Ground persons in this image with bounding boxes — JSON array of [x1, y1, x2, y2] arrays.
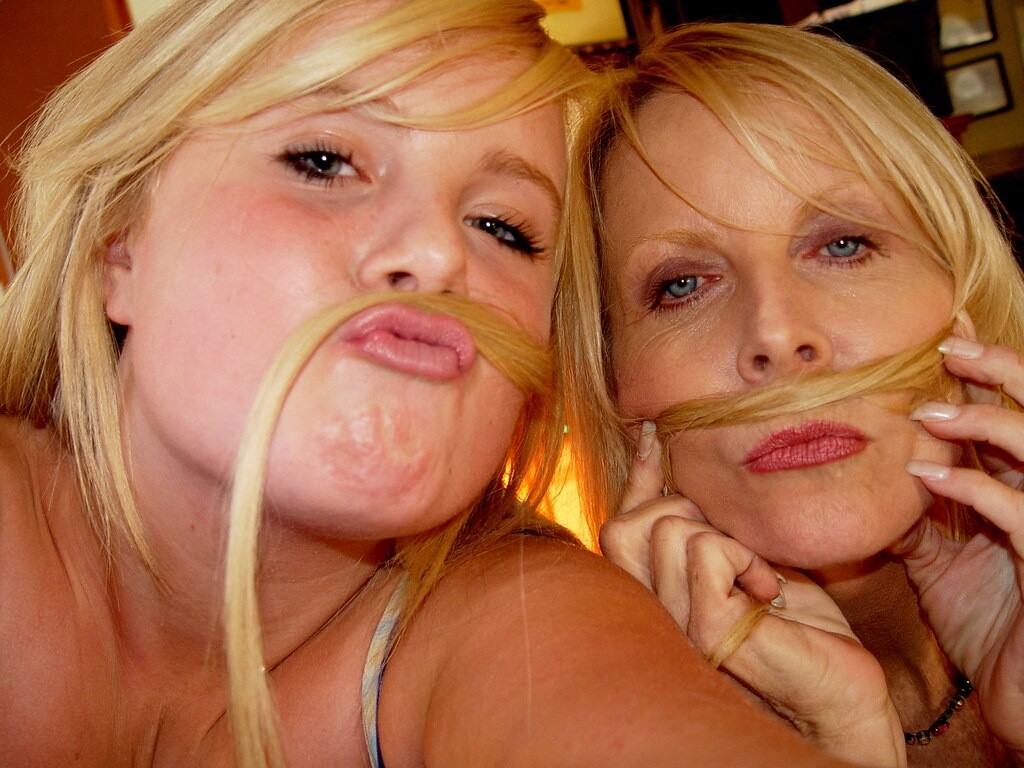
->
[[0, 0, 864, 767], [552, 22, 1024, 767]]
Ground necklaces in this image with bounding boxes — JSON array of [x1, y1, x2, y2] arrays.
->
[[904, 678, 972, 747]]
